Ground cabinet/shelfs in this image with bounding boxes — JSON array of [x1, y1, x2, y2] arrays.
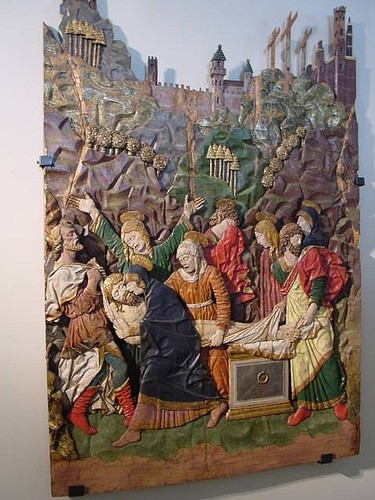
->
[[225, 353, 294, 421]]
